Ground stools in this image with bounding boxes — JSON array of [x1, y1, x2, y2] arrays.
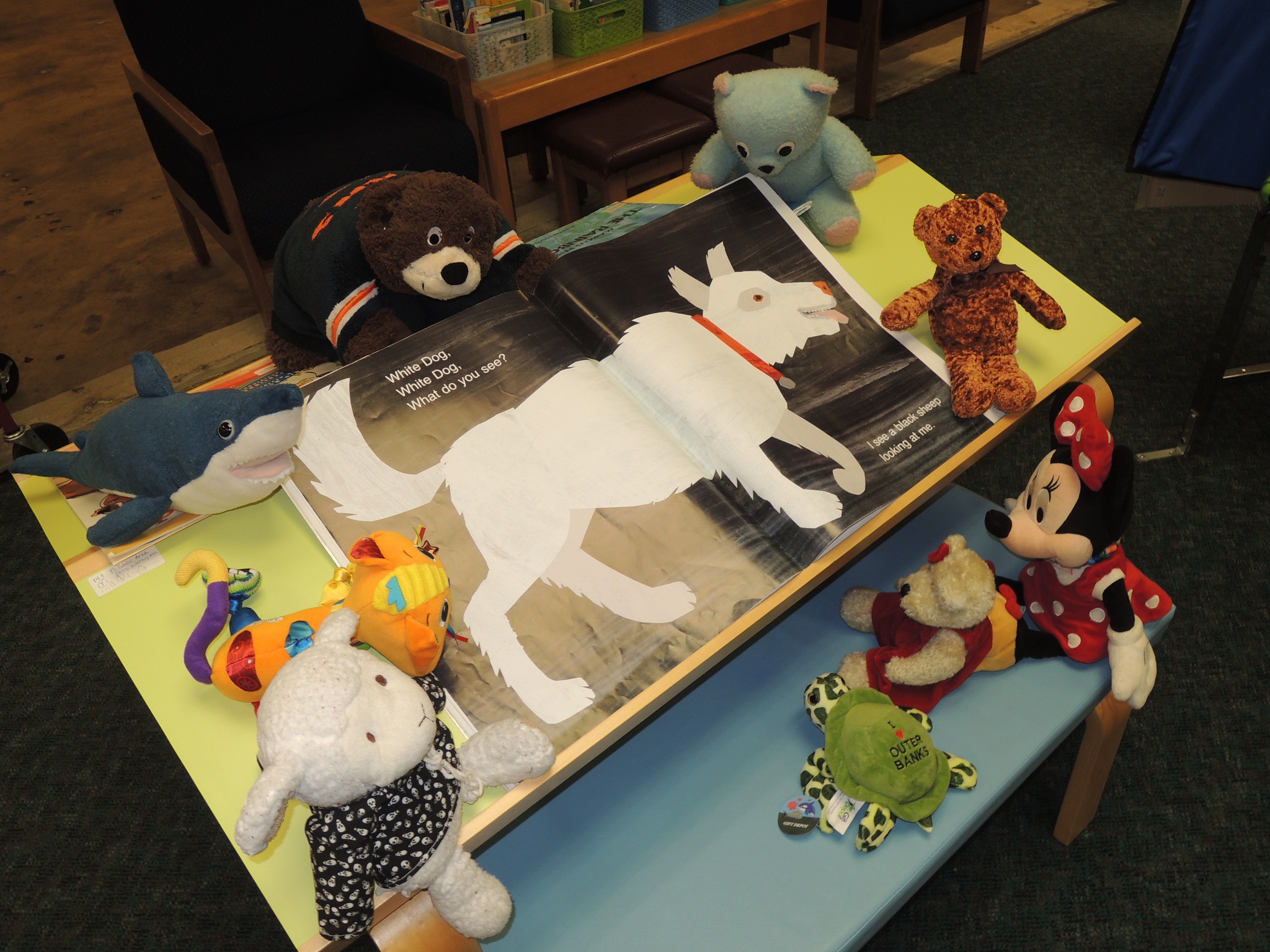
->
[[654, 53, 786, 121], [546, 87, 713, 228]]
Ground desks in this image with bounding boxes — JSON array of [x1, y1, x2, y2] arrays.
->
[[11, 154, 1150, 952], [473, 0, 828, 242]]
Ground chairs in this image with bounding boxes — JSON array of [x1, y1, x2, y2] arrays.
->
[[791, 0, 991, 122], [113, 0, 491, 327]]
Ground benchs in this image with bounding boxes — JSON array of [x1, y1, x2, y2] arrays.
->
[[477, 481, 1175, 951]]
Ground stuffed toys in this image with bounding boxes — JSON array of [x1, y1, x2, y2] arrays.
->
[[973, 383, 1157, 711], [237, 608, 557, 940], [172, 528, 453, 712], [263, 167, 561, 374], [800, 673, 980, 849], [832, 533, 996, 715], [687, 67, 873, 247], [881, 191, 1066, 420], [8, 349, 301, 546]]
